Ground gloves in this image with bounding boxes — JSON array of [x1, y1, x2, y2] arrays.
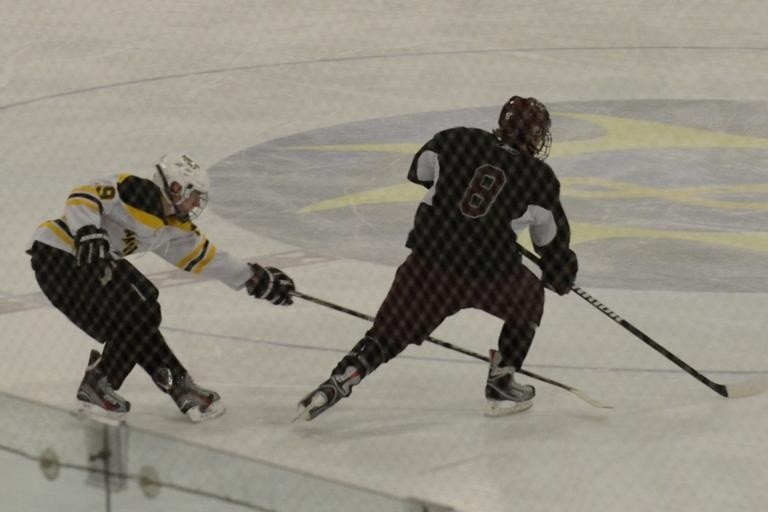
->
[[245, 261, 295, 306], [73, 223, 112, 269], [538, 249, 578, 294]]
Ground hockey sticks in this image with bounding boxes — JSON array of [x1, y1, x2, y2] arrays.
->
[[292, 291, 614, 409], [520, 242, 767, 399]]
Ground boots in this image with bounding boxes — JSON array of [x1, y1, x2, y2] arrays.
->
[[485, 349, 535, 403], [77, 349, 130, 413], [296, 365, 361, 422], [150, 366, 220, 415]]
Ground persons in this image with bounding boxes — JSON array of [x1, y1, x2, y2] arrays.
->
[[26, 149, 294, 414], [297, 94, 579, 420]]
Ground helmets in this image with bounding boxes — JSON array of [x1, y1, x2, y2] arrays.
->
[[153, 151, 209, 224], [497, 96, 552, 162]]
